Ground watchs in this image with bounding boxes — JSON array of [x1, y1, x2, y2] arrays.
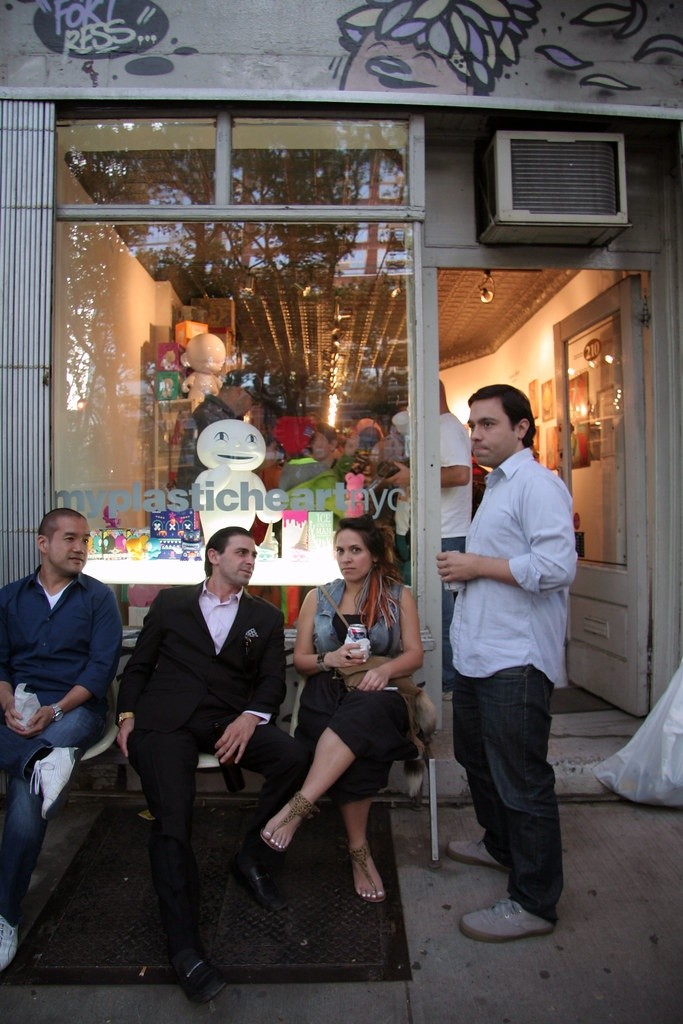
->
[[50, 704, 63, 721], [118, 712, 134, 725]]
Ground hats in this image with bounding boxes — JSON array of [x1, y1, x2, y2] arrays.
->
[[315, 422, 337, 443], [222, 369, 274, 401]]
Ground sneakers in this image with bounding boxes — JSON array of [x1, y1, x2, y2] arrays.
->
[[0, 914, 19, 972], [29, 745, 80, 820]]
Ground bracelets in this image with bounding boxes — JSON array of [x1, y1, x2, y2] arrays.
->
[[317, 652, 331, 671]]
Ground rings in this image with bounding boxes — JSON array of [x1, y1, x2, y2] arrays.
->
[[346, 652, 352, 660]]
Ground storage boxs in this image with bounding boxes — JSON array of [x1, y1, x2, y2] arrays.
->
[[208, 327, 230, 375], [156, 342, 187, 375], [208, 298, 235, 335], [175, 321, 209, 349], [156, 371, 186, 400]]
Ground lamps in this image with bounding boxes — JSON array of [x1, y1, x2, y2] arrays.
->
[[478, 271, 495, 304], [386, 286, 400, 297], [244, 275, 254, 292], [325, 303, 353, 395], [292, 282, 311, 296]]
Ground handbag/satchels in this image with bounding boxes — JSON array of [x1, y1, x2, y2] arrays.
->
[[337, 654, 405, 691]]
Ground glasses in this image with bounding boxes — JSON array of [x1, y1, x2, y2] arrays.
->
[[245, 387, 260, 407]]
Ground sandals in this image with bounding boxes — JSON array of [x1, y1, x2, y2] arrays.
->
[[345, 840, 386, 904], [260, 791, 321, 853]]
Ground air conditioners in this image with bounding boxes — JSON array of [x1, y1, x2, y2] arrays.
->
[[474, 130, 629, 247]]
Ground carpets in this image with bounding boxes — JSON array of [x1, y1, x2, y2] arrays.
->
[[0, 797, 416, 986]]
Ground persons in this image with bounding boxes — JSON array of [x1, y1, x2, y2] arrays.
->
[[253, 415, 384, 628], [182, 333, 226, 410], [435, 385, 575, 941], [385, 380, 473, 694], [115, 528, 299, 1006], [174, 368, 263, 510], [0, 508, 123, 970], [260, 517, 424, 903]]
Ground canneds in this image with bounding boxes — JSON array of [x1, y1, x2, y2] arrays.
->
[[348, 624, 367, 642], [443, 550, 466, 590]]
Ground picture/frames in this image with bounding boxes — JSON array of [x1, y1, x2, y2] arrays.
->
[[529, 370, 592, 472]]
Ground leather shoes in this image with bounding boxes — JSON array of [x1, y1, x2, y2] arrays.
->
[[232, 852, 288, 912], [170, 948, 226, 1005]]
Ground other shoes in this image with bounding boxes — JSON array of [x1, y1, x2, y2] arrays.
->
[[446, 839, 515, 873], [459, 897, 553, 943]]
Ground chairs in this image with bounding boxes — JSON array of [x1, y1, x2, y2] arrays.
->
[[0, 665, 442, 870]]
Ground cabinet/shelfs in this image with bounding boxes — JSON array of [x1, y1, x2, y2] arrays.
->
[[155, 399, 198, 492]]
